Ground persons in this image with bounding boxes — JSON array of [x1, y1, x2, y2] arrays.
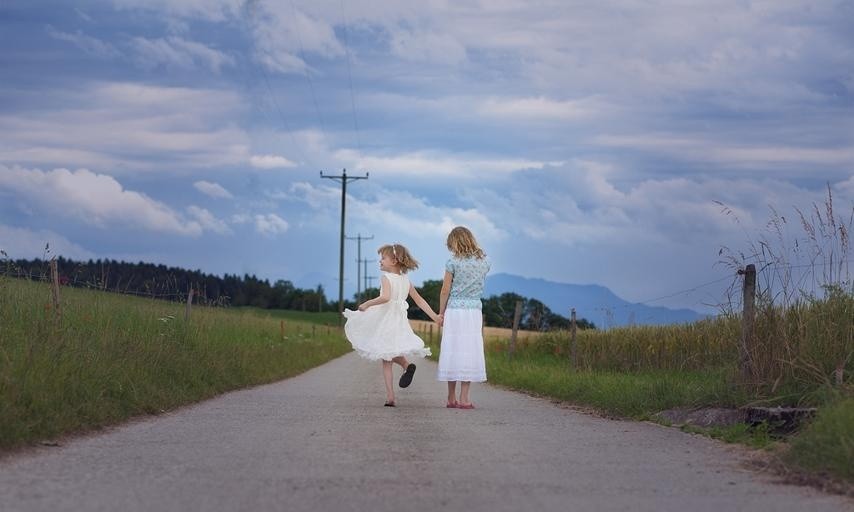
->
[[341, 244, 444, 407], [438, 226, 491, 409]]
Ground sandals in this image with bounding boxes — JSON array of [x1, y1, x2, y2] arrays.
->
[[398, 363, 416, 388], [384, 401, 395, 406], [447, 399, 475, 409]]
